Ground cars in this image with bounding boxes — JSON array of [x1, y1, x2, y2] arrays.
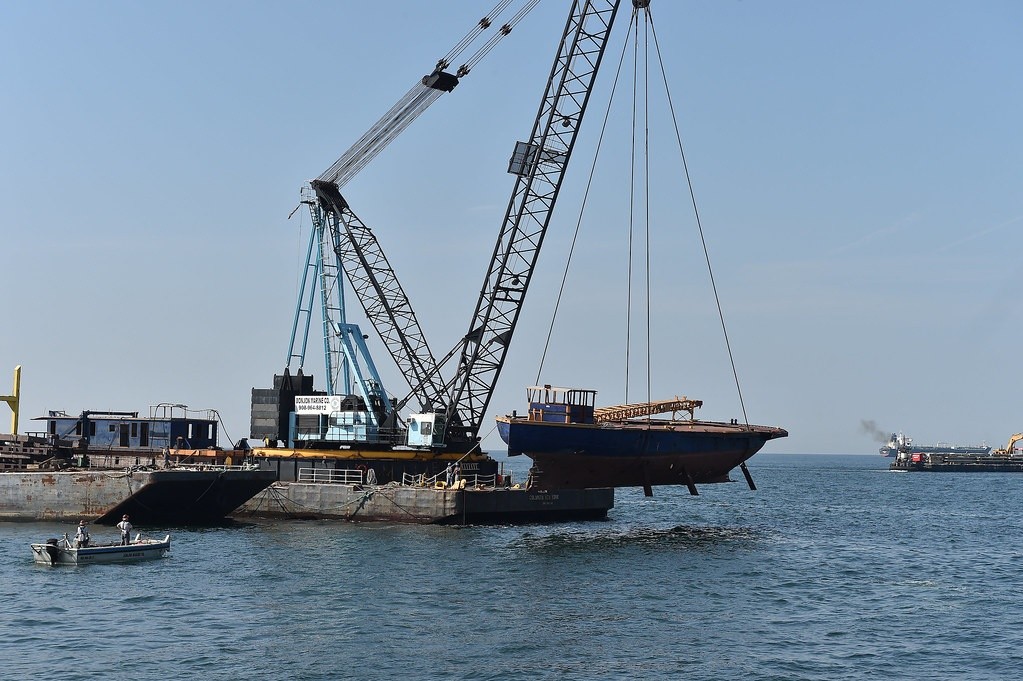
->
[[131, 463, 228, 473]]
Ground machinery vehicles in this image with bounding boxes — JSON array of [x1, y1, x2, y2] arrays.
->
[[991, 431, 1023, 457], [279, 1, 654, 452]]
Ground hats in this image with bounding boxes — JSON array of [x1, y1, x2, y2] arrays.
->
[[122, 515, 129, 521], [79, 520, 85, 525]]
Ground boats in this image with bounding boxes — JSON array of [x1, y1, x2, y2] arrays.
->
[[31, 532, 170, 567], [496, 384, 788, 490], [878, 430, 993, 458]]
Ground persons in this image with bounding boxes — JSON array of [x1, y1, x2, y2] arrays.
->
[[446, 462, 461, 488], [262, 434, 270, 448], [117, 514, 133, 547], [164, 445, 172, 469], [76, 519, 90, 548]]
